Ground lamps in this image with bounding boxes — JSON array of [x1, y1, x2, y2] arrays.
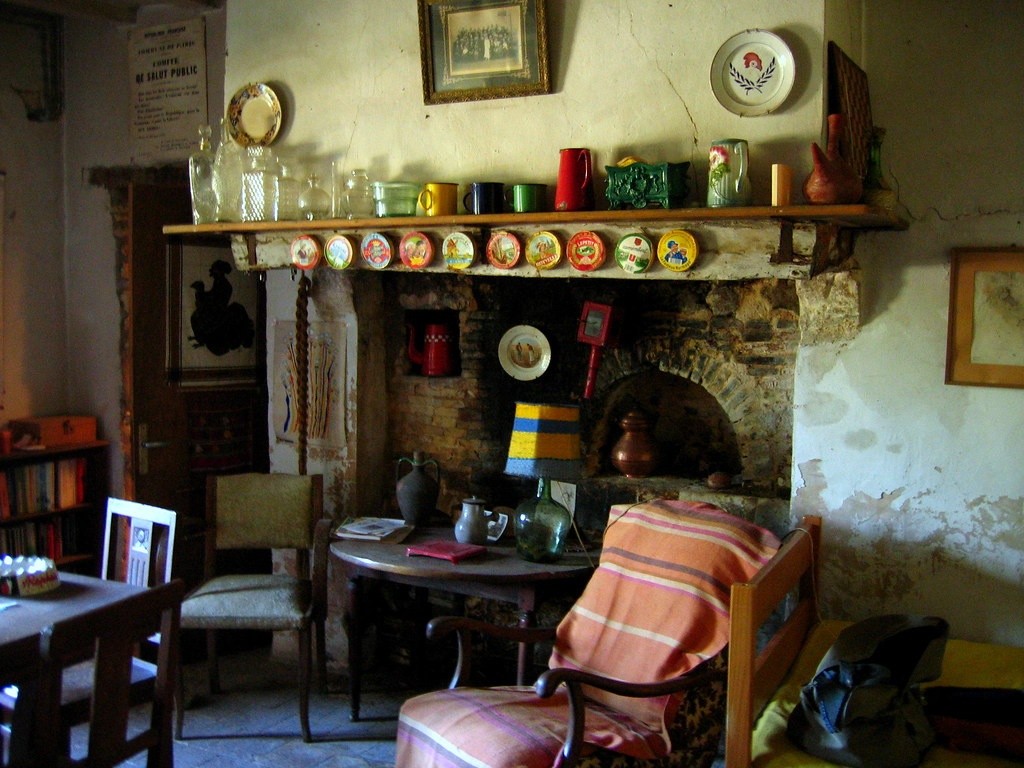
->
[[502, 402, 583, 563]]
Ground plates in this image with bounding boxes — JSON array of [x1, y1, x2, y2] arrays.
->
[[498, 324, 551, 381], [226, 83, 282, 148], [709, 28, 796, 118]]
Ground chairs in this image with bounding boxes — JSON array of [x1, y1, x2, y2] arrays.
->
[[0, 497, 183, 768], [394, 499, 782, 768], [176, 474, 325, 743]]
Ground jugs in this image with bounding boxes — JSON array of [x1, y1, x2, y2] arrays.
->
[[406, 324, 455, 377], [553, 148, 594, 211]]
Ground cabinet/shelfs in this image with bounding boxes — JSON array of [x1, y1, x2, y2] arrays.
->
[[0, 439, 113, 580]]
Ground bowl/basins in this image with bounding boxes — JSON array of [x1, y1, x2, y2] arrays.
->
[[371, 181, 419, 218]]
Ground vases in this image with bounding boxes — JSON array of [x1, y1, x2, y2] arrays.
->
[[340, 169, 375, 219]]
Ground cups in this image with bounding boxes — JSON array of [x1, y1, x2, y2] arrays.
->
[[419, 182, 459, 216], [506, 183, 547, 212], [707, 138, 750, 208], [462, 182, 504, 214]]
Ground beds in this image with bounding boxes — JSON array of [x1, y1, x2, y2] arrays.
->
[[725, 514, 1024, 768]]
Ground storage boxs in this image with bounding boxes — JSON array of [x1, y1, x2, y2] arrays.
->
[[9, 414, 97, 446]]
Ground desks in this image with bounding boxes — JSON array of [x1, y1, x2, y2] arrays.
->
[[0, 570, 158, 692], [327, 520, 601, 723]]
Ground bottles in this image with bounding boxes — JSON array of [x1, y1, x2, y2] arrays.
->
[[189, 125, 216, 225], [612, 407, 656, 477], [455, 495, 487, 545], [395, 446, 440, 529], [299, 161, 374, 220], [241, 145, 279, 222], [275, 164, 300, 220], [211, 118, 243, 223]]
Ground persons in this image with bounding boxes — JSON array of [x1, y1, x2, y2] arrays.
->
[[454, 25, 512, 61]]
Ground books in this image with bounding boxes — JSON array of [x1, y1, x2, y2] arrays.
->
[[336, 516, 381, 540], [0, 459, 85, 560]]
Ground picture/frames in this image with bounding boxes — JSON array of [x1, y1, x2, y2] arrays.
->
[[416, 0, 553, 107], [943, 244, 1024, 389]]
